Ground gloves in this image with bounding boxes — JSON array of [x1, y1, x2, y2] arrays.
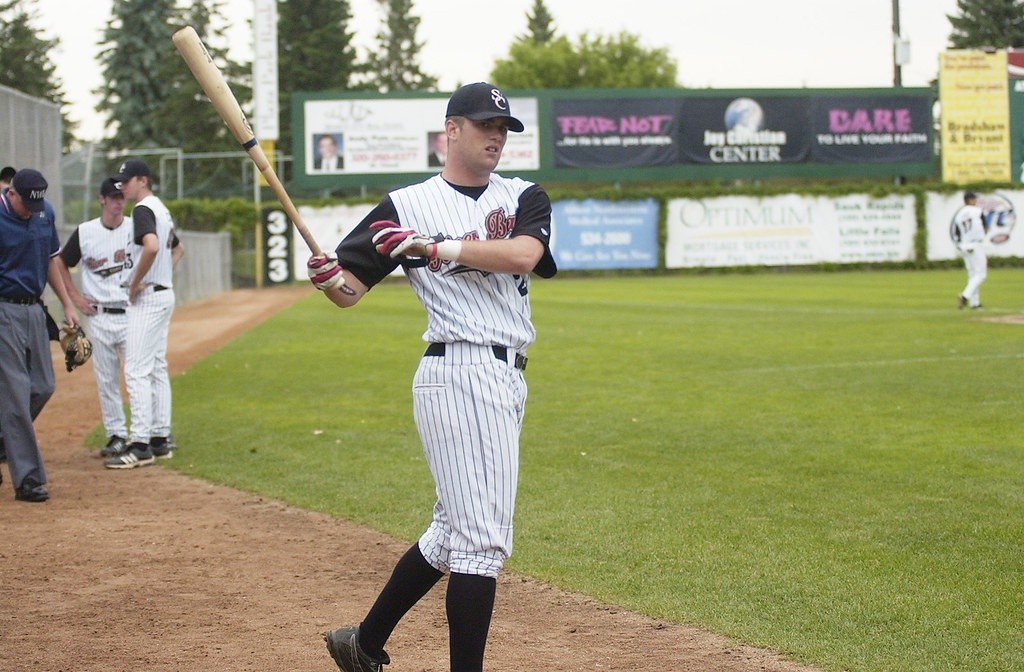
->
[[307, 253, 345, 291], [369, 219, 438, 259]]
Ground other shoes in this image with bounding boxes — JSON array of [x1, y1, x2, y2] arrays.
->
[[970, 304, 983, 310], [15, 477, 49, 501], [958, 294, 968, 309]]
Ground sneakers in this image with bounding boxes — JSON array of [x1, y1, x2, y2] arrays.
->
[[150, 439, 176, 460], [105, 442, 154, 469], [321, 621, 390, 672], [101, 435, 126, 457]]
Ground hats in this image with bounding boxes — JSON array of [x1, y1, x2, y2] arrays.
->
[[101, 178, 123, 198], [13, 168, 48, 212], [110, 159, 151, 182], [446, 82, 524, 133]]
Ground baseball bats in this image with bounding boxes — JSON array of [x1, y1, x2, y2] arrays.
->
[[163, 21, 332, 266]]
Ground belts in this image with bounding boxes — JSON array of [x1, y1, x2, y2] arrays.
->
[[424, 342, 528, 370], [0, 295, 41, 306], [154, 285, 168, 292], [91, 305, 125, 314]]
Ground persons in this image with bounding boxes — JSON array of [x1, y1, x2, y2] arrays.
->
[[428, 132, 448, 167], [314, 135, 344, 169], [0, 166, 80, 502], [307, 82, 558, 672], [103, 160, 187, 470], [56, 176, 178, 458], [950, 192, 989, 310]]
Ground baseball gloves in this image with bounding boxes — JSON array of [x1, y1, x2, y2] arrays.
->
[[58, 320, 92, 365]]
